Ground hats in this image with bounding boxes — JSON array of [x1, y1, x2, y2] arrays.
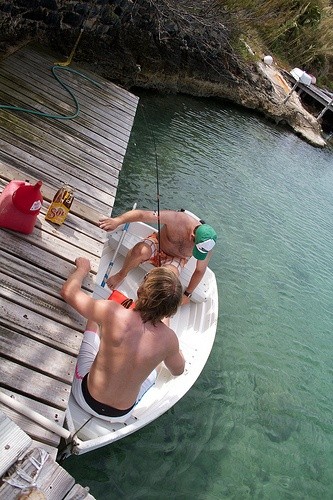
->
[[192, 224, 217, 260]]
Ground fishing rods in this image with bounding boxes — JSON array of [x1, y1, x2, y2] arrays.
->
[[138, 102, 162, 267]]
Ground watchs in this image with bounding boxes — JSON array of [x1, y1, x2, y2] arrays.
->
[[183, 290, 193, 299]]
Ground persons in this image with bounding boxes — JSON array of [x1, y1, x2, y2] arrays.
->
[[98, 208, 217, 307], [61, 256, 187, 424]]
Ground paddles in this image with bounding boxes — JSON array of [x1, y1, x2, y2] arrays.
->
[[100, 201, 138, 288]]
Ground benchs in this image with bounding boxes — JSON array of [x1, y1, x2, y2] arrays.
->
[[108, 230, 210, 304], [68, 389, 140, 442]]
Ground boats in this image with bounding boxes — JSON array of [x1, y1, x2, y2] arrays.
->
[[47, 215, 220, 457]]
